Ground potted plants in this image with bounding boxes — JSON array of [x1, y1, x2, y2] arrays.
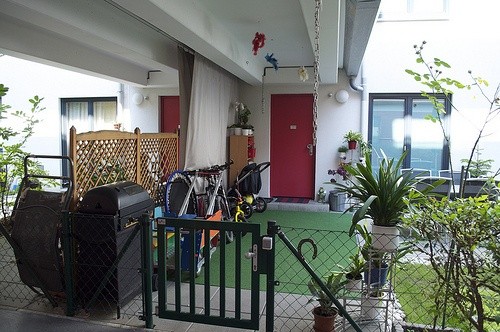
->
[[303, 144, 446, 332], [343, 130, 362, 149], [338, 146, 349, 158], [227, 122, 254, 136]]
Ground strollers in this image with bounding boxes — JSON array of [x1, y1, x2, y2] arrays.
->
[[224, 161, 272, 219]]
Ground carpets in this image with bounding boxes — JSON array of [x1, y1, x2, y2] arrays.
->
[[150, 208, 364, 301]]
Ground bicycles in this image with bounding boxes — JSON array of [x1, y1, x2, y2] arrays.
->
[[180, 159, 236, 278]]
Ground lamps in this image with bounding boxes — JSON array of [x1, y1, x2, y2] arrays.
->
[[335, 89, 349, 104], [132, 93, 143, 104]]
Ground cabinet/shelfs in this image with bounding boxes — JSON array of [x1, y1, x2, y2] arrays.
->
[[227, 135, 254, 190]]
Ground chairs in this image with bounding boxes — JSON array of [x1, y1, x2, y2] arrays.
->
[[400, 168, 498, 212]]
